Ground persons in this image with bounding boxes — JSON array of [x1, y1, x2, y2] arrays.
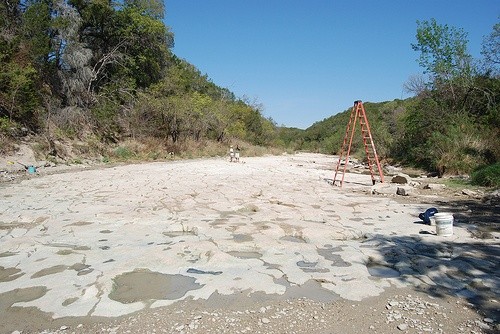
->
[[228, 144, 234, 162], [235, 144, 241, 162]]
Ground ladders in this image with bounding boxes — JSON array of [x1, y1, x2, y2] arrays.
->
[[331, 100, 385, 188]]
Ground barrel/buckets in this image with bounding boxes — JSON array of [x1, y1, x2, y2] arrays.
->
[[434, 212, 454, 236]]
